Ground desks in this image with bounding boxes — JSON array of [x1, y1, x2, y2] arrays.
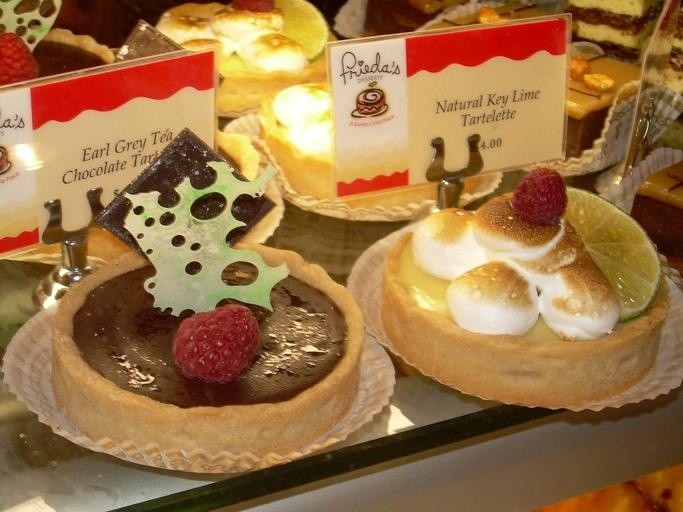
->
[[566, 56, 642, 156]]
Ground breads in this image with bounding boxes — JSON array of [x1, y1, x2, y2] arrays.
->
[[156, 1, 339, 116]]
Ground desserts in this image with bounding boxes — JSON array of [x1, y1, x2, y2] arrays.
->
[[596, 148, 682, 274], [334, 1, 447, 39]]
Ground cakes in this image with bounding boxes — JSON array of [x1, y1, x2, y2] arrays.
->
[[409, 0, 682, 175], [347, 168, 683, 412], [1, 1, 115, 87], [223, 83, 503, 221], [3, 127, 395, 472]]
